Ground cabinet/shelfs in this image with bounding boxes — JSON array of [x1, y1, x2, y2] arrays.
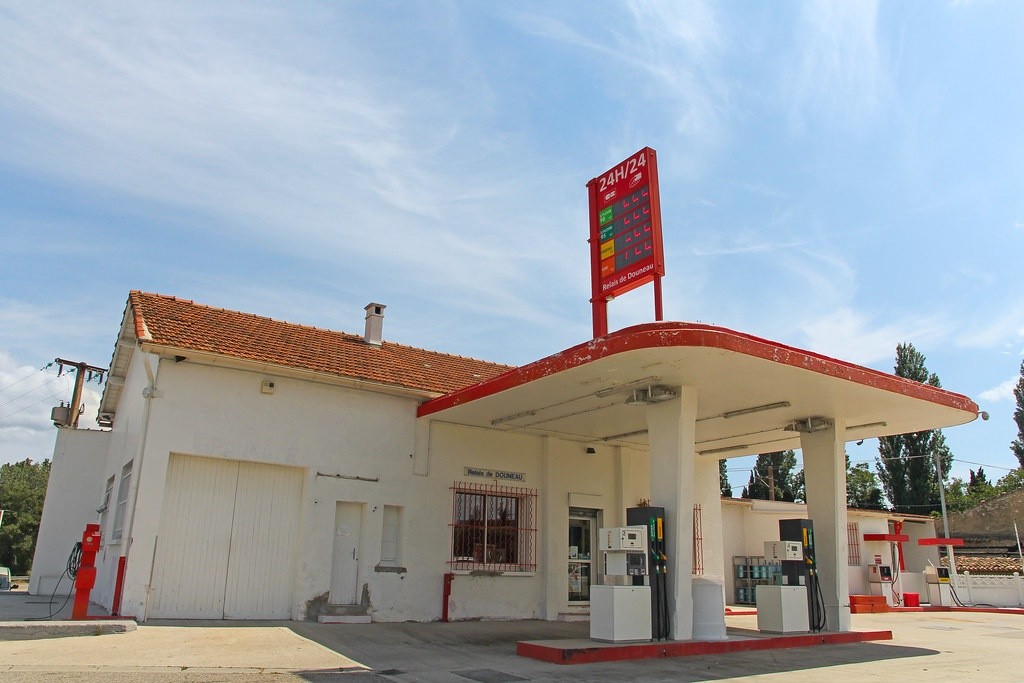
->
[[733, 555, 781, 604]]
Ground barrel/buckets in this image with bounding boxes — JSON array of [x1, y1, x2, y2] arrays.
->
[[903, 593, 919, 607]]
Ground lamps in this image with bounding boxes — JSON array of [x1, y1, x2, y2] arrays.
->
[[783, 417, 831, 432], [623, 385, 679, 406]]
